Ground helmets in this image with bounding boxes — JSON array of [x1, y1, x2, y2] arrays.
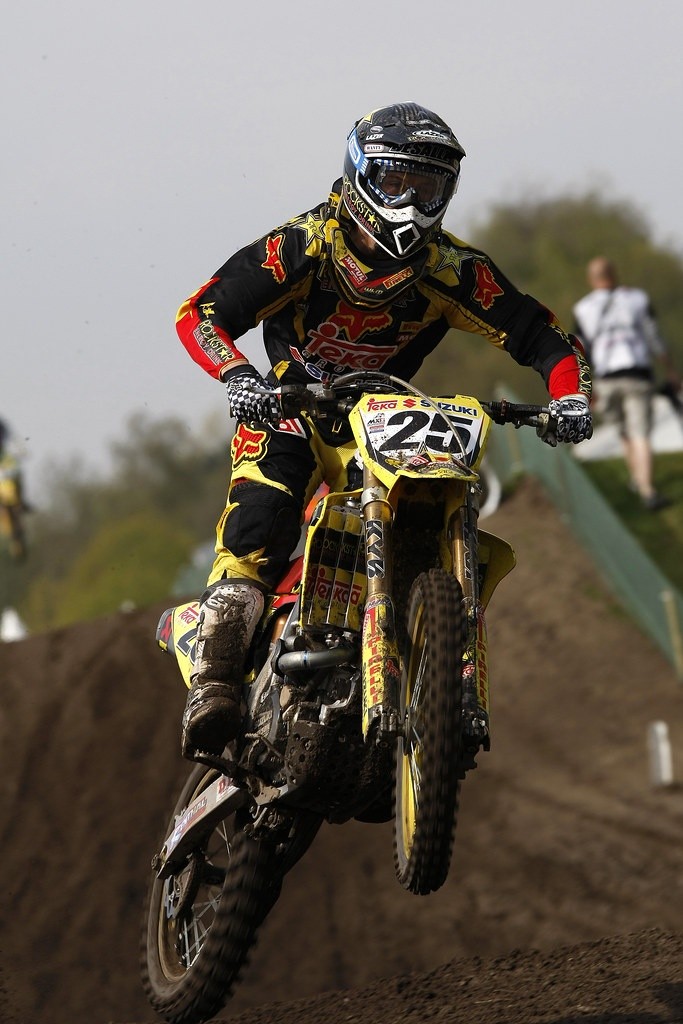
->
[[343, 102, 464, 258]]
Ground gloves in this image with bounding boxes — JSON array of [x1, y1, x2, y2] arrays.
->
[[540, 400, 593, 448], [225, 374, 281, 424]]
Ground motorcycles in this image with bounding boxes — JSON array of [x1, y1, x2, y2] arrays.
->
[[133, 364, 562, 1022]]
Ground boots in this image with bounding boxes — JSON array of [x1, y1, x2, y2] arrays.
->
[[180, 579, 270, 763]]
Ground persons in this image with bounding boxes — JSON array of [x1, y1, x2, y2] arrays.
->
[[571, 256, 665, 506], [0, 416, 33, 644], [177, 105, 592, 760]]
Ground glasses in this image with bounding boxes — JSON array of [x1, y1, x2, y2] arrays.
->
[[351, 154, 460, 211]]
